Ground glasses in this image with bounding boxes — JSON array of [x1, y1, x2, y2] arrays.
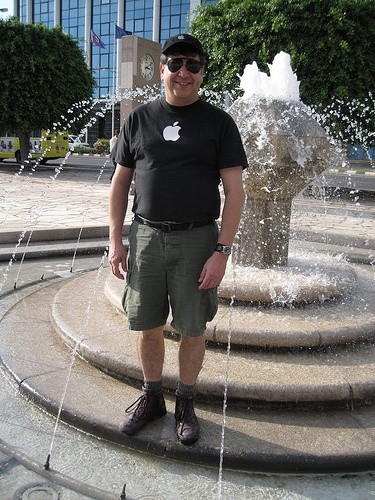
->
[[165, 58, 202, 74]]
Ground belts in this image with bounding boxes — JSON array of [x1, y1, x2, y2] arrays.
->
[[135, 214, 214, 233]]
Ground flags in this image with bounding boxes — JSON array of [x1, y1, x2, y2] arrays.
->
[[115, 25, 132, 38], [91, 29, 106, 49]]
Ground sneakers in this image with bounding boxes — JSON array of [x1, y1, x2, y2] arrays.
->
[[120, 387, 167, 434], [176, 399, 199, 445]]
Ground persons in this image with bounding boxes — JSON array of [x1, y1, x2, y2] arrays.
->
[[109, 129, 119, 182], [109, 34, 250, 444]]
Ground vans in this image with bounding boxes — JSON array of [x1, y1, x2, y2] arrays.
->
[[68, 135, 90, 153]]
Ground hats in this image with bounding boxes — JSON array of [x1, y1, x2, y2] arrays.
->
[[161, 33, 204, 57]]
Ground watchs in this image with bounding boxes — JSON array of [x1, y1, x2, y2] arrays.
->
[[215, 243, 231, 256]]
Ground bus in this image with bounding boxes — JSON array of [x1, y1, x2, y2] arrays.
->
[[0, 128, 71, 164]]
